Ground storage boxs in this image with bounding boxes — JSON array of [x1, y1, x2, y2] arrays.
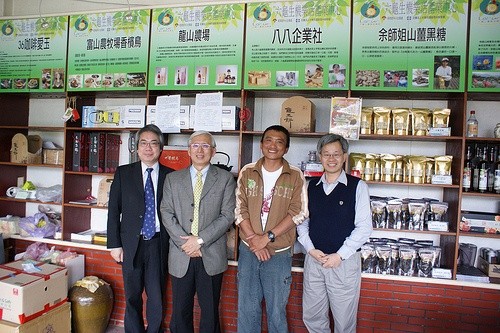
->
[[478, 256, 500, 277], [82, 105, 145, 128], [0, 302, 72, 333], [15, 252, 84, 289], [190, 106, 240, 130], [146, 105, 190, 129], [460, 212, 500, 234], [0, 259, 69, 323]]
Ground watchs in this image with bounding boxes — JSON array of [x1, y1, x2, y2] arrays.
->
[[267, 230, 275, 242], [340, 257, 345, 261], [197, 236, 204, 247]]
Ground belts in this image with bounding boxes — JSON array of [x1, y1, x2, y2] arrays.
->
[[140, 232, 159, 240]]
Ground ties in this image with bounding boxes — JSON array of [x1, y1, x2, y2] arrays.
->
[[191, 171, 203, 237], [142, 168, 156, 240]]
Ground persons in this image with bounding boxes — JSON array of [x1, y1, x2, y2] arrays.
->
[[106, 123, 176, 333], [159, 131, 240, 333], [296, 133, 373, 333], [234, 125, 309, 333], [273, 48, 500, 93]]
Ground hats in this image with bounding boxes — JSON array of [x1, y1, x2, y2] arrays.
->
[[441, 57, 450, 62]]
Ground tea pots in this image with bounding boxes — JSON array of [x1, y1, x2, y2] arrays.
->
[[210, 151, 234, 172]]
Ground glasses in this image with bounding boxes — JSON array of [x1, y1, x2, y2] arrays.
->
[[188, 143, 214, 150], [137, 141, 160, 147], [320, 153, 344, 159]]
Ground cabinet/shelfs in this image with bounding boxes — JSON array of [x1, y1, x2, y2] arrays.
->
[[0, 89, 500, 280]]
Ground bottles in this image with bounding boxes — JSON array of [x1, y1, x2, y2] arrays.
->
[[462, 144, 500, 194], [67, 276, 113, 333], [466, 110, 479, 139]]
